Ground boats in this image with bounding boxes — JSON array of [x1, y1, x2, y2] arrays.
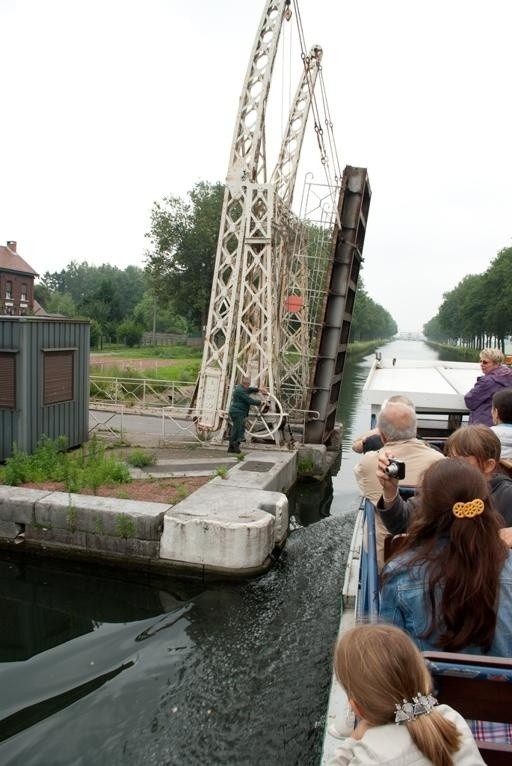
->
[[318, 348, 512, 766]]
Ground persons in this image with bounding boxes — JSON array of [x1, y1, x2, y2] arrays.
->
[[377, 454, 511, 660], [463, 347, 512, 428], [486, 388, 511, 461], [226, 377, 270, 454], [323, 622, 490, 766], [351, 395, 424, 454], [353, 401, 446, 576], [373, 423, 512, 549]]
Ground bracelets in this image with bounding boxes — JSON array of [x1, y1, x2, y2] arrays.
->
[[382, 485, 400, 504]]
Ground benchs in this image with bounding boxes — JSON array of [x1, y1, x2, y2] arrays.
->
[[381, 436, 512, 766]]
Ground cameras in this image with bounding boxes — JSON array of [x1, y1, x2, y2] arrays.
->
[[383, 457, 406, 481]]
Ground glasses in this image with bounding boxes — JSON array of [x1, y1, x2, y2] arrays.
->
[[479, 360, 494, 364]]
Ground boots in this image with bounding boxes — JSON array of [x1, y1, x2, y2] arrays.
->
[[228, 441, 242, 455]]
